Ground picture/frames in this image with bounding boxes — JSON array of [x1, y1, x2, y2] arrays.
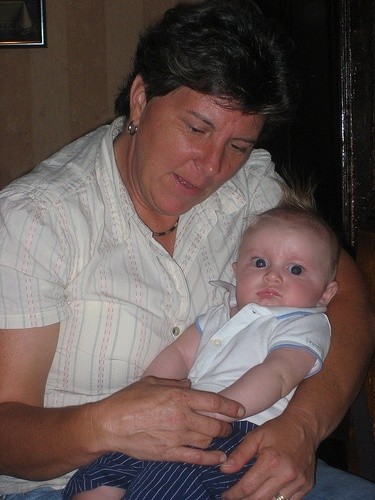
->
[[0, 0, 48, 48]]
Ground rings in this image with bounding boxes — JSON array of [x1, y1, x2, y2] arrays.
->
[[275, 493, 288, 500]]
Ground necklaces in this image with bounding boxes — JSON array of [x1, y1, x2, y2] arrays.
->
[[112, 133, 178, 236]]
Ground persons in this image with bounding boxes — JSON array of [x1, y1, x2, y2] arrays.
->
[[2, 0, 375, 500], [64, 185, 339, 498]]
